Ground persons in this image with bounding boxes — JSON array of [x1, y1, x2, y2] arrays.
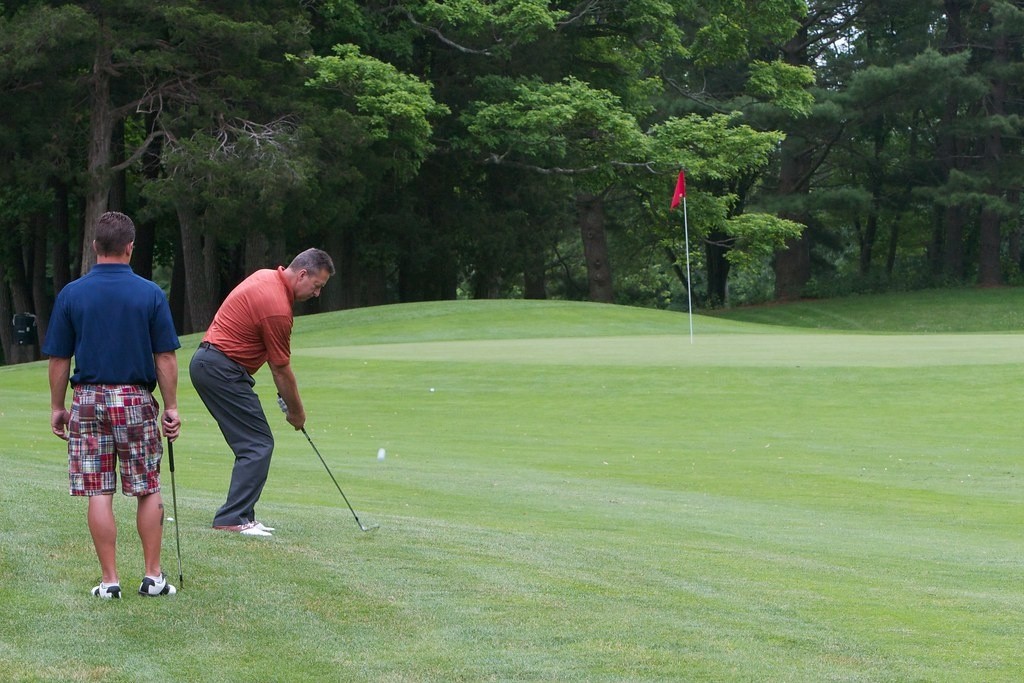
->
[[189, 248, 335, 535], [39, 213, 181, 599]]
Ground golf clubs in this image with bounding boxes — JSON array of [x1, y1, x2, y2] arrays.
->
[[276, 391, 381, 532], [165, 416, 183, 588]]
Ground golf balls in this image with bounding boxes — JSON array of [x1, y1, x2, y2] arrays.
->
[[375, 448, 386, 462], [429, 388, 435, 392]]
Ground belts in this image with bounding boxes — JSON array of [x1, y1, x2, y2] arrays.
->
[[198, 342, 222, 353]]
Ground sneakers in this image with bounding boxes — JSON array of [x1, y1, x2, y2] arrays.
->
[[212, 521, 276, 537], [91, 583, 123, 601], [139, 573, 179, 597]]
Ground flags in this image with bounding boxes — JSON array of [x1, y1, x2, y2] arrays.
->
[[670, 171, 684, 208]]
